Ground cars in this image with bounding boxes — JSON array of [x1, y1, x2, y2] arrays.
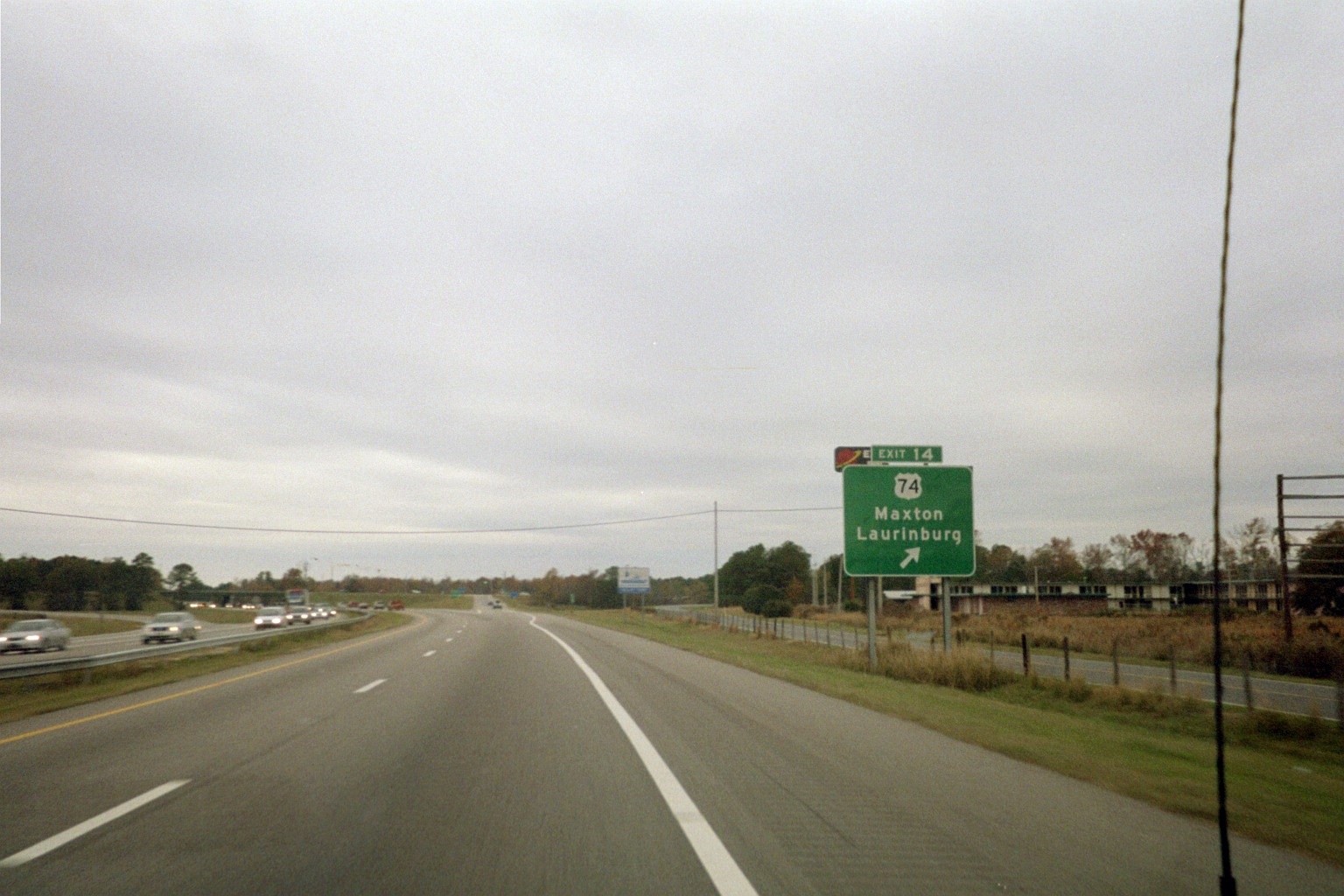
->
[[287, 606, 313, 626], [346, 598, 406, 612], [488, 598, 502, 609], [186, 600, 255, 610], [140, 612, 202, 645], [310, 604, 331, 621], [0, 619, 73, 655], [253, 606, 290, 631], [329, 606, 337, 617]]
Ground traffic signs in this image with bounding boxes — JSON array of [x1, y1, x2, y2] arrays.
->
[[842, 463, 976, 578]]
